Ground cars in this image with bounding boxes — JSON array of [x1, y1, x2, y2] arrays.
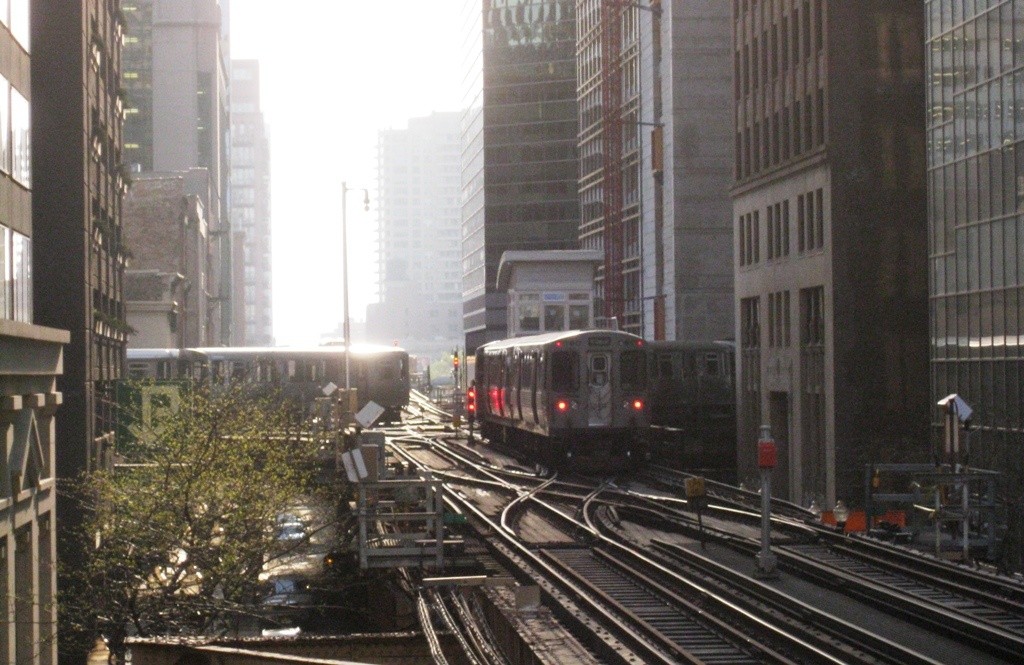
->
[[254, 573, 321, 634], [274, 514, 309, 551]]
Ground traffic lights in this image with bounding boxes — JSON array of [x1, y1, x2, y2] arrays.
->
[[467, 379, 478, 447], [454, 352, 458, 387]]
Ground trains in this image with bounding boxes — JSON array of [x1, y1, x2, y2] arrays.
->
[[474, 328, 737, 479], [126, 346, 410, 427]]
